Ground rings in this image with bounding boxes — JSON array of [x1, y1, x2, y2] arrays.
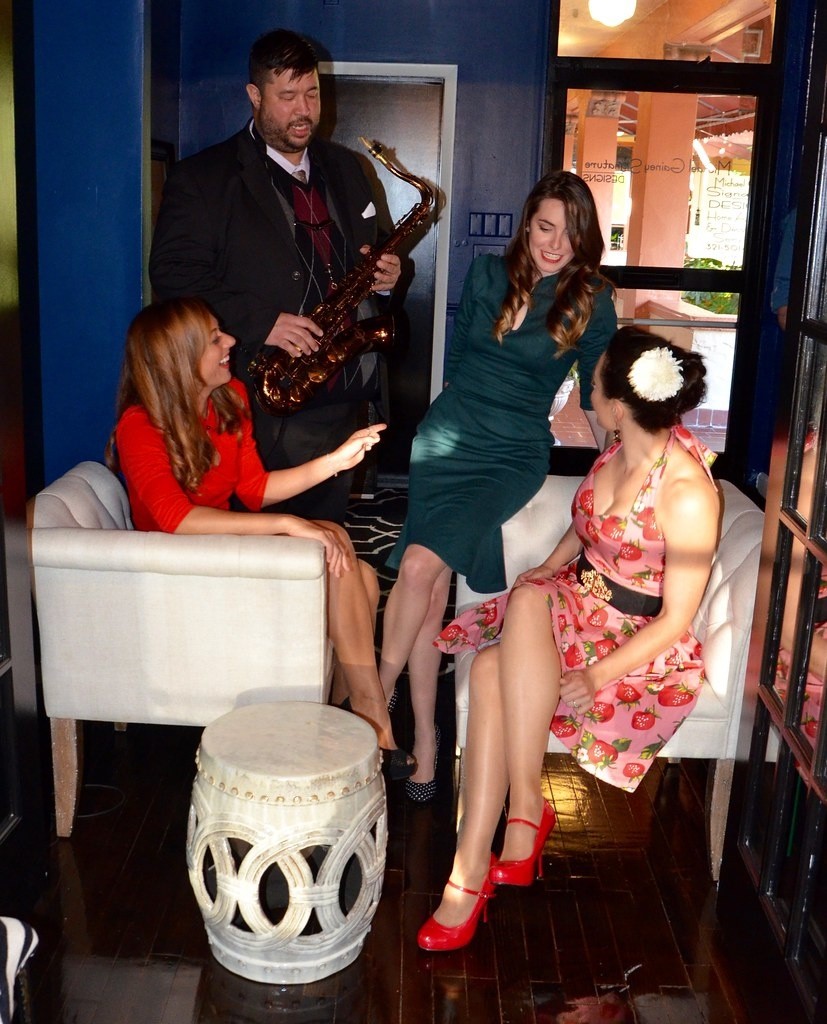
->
[[573, 701, 578, 708]]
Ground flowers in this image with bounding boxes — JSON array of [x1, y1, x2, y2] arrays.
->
[[627, 344, 684, 403]]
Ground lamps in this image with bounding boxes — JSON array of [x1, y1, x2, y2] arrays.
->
[[588, 0, 637, 28]]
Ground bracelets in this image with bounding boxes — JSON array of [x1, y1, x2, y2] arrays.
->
[[327, 452, 338, 478]]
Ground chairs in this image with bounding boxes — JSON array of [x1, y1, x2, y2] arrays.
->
[[453, 475, 767, 892], [26, 461, 334, 838]]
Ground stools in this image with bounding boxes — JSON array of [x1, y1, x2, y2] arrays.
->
[[186, 700, 389, 985]]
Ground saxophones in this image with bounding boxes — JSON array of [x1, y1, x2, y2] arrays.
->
[[245, 137, 436, 416]]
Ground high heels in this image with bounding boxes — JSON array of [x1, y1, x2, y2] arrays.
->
[[386, 687, 399, 712], [418, 850, 499, 952], [405, 724, 442, 803], [489, 798, 556, 886]]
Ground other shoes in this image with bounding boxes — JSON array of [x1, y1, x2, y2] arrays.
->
[[379, 746, 417, 784]]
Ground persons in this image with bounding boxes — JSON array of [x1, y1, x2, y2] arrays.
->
[[374, 172, 623, 802], [115, 302, 419, 778], [157, 33, 407, 504], [417, 325, 721, 948]]
